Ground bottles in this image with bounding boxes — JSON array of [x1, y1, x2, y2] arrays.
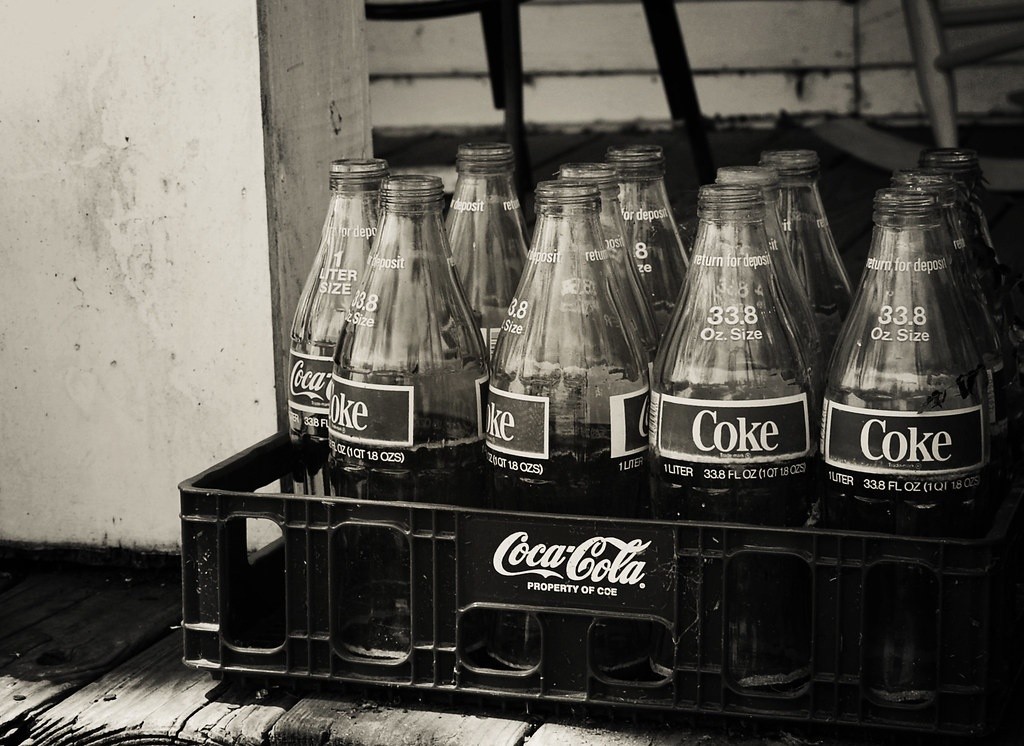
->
[[287, 158, 390, 496], [820, 149, 1024, 701], [484, 143, 696, 667], [328, 176, 486, 656], [759, 151, 854, 366], [445, 143, 532, 362], [651, 185, 819, 685], [716, 166, 825, 399]]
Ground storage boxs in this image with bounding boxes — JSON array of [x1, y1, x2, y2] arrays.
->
[[178, 428, 1024, 743]]
